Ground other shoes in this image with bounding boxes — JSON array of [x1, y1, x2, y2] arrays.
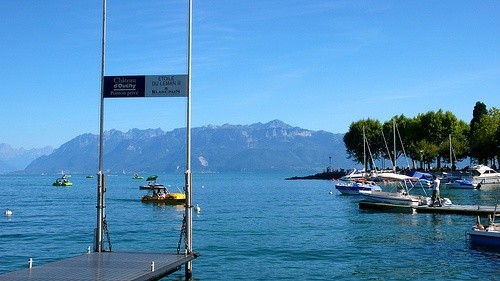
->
[[437, 205, 441, 207], [428, 205, 433, 207]]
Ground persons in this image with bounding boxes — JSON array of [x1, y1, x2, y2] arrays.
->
[[452, 163, 456, 171], [62, 174, 67, 181], [428, 175, 442, 207], [153, 191, 161, 198], [148, 182, 152, 187]]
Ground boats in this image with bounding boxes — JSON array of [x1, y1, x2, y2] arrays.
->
[[133, 172, 144, 180], [86, 175, 94, 178], [138, 174, 166, 190], [53, 181, 73, 186], [334, 118, 500, 250], [141, 192, 187, 205]]
